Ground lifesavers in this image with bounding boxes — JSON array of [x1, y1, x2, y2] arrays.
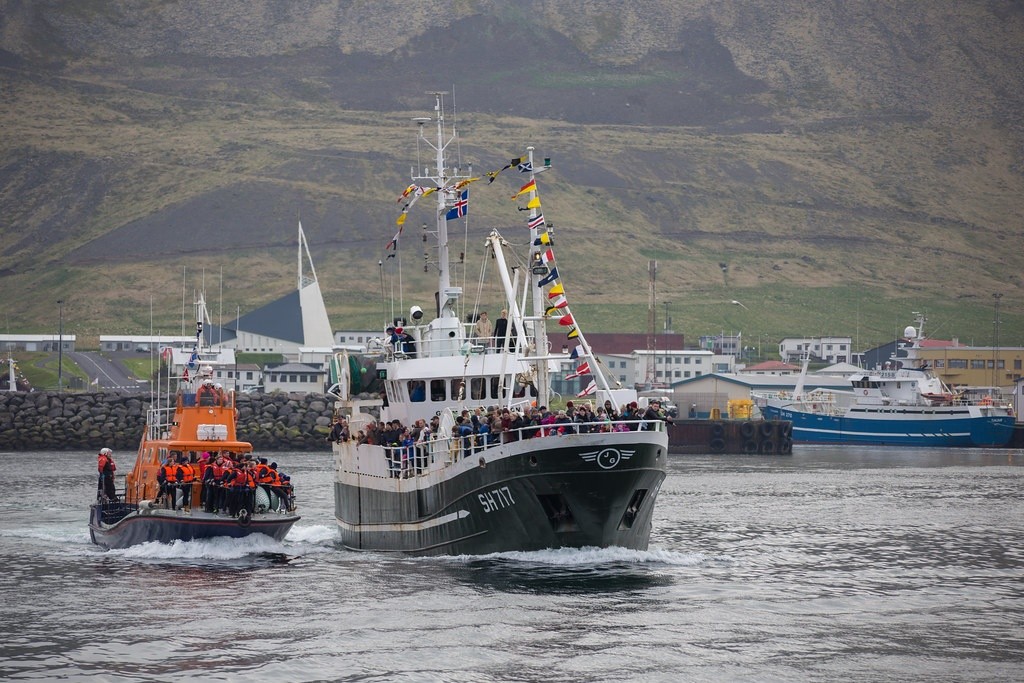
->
[[863, 390, 868, 395], [709, 422, 728, 453], [741, 422, 792, 454]]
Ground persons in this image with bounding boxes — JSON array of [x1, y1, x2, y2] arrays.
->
[[154, 450, 295, 519], [493, 309, 511, 353], [195, 379, 217, 407], [326, 400, 676, 478], [475, 312, 493, 354], [214, 383, 228, 406], [386, 327, 415, 360], [98, 448, 119, 503], [509, 318, 527, 352]]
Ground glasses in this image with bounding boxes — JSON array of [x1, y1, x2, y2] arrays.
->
[[606, 404, 610, 405]]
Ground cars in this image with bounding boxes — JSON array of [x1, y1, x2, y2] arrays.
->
[[135, 346, 151, 353]]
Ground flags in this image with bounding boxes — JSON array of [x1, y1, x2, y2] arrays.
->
[[90, 378, 98, 385], [0, 358, 35, 392], [385, 179, 469, 260], [486, 155, 598, 398], [162, 321, 203, 381]]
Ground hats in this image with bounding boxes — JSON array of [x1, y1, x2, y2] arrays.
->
[[181, 458, 188, 464], [328, 423, 334, 427], [431, 416, 439, 420], [202, 452, 210, 459]]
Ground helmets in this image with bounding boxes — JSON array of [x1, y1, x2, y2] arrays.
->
[[99, 448, 108, 456]]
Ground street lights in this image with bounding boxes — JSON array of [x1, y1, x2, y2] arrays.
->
[[58, 300, 64, 379], [663, 301, 672, 355], [991, 292, 1004, 386]]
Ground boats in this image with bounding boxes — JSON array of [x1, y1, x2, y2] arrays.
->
[[920, 392, 951, 402], [330, 84, 668, 553], [749, 310, 1017, 449], [87, 266, 301, 551]]
